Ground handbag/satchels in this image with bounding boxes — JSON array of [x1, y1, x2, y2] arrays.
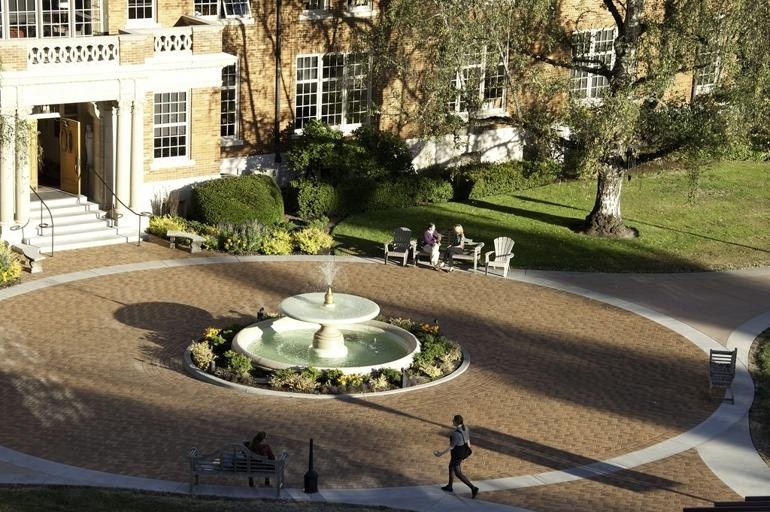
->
[[389, 244, 405, 252], [464, 443, 472, 459], [417, 240, 425, 251]]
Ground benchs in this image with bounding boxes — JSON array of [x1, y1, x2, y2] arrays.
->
[[186, 447, 288, 499], [708, 347, 737, 405], [411, 234, 485, 271], [167, 230, 206, 254]]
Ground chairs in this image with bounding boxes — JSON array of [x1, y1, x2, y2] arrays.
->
[[384, 226, 412, 266], [485, 236, 515, 278]]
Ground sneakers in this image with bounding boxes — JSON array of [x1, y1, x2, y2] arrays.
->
[[266, 478, 270, 485], [249, 480, 254, 486], [441, 485, 453, 492], [431, 262, 454, 273], [472, 487, 479, 499]]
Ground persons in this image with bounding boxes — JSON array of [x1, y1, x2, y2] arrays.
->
[[437, 415, 480, 499], [440, 224, 464, 271], [247, 432, 275, 487], [422, 222, 442, 270]]
